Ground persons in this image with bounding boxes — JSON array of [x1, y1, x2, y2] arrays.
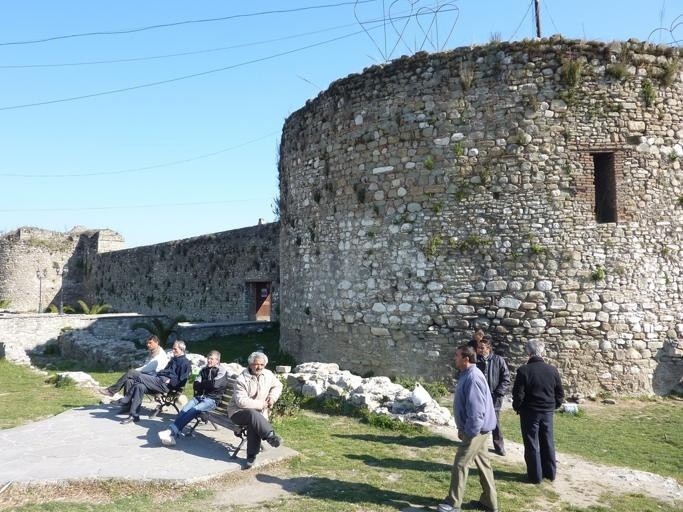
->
[[99, 334, 168, 413], [157, 349, 228, 447], [467, 330, 484, 350], [474, 335, 512, 457], [109, 340, 191, 426], [511, 338, 564, 484], [225, 350, 284, 470], [435, 343, 504, 512]]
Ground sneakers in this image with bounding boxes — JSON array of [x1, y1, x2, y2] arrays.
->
[[471, 499, 498, 512], [246, 455, 257, 468], [438, 503, 460, 512], [498, 449, 506, 456], [158, 427, 178, 446], [266, 431, 283, 447]]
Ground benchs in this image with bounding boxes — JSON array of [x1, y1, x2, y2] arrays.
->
[[190, 378, 264, 460], [147, 379, 188, 419]]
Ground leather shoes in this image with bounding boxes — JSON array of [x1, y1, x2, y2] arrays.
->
[[99, 386, 140, 424]]
